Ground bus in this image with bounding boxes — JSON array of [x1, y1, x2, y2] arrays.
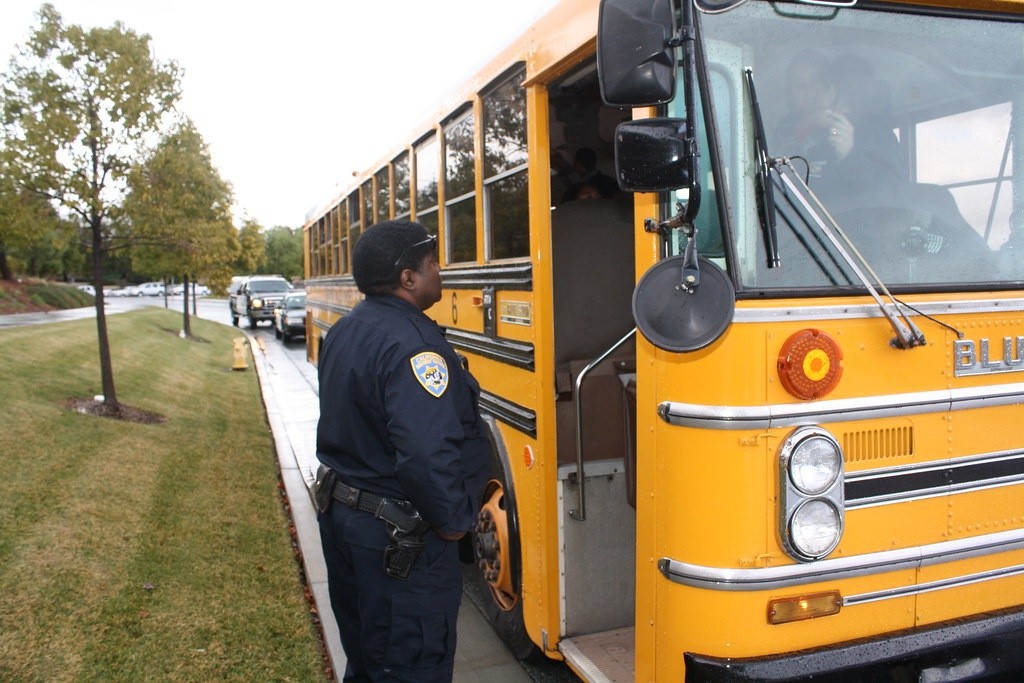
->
[[302, 0, 1024, 683]]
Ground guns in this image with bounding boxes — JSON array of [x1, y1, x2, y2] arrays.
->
[[374, 497, 422, 579]]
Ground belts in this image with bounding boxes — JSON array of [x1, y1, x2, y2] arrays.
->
[[315, 467, 407, 517]]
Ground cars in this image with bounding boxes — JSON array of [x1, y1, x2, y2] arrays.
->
[[273, 291, 307, 345], [78, 281, 212, 297]]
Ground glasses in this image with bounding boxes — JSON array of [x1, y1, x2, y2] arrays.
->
[[396, 235, 438, 268]]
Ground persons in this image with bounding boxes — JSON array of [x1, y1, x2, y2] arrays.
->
[[769, 49, 911, 214], [314, 220, 480, 683]]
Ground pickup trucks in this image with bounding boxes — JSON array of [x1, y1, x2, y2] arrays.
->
[[228, 277, 296, 328]]
